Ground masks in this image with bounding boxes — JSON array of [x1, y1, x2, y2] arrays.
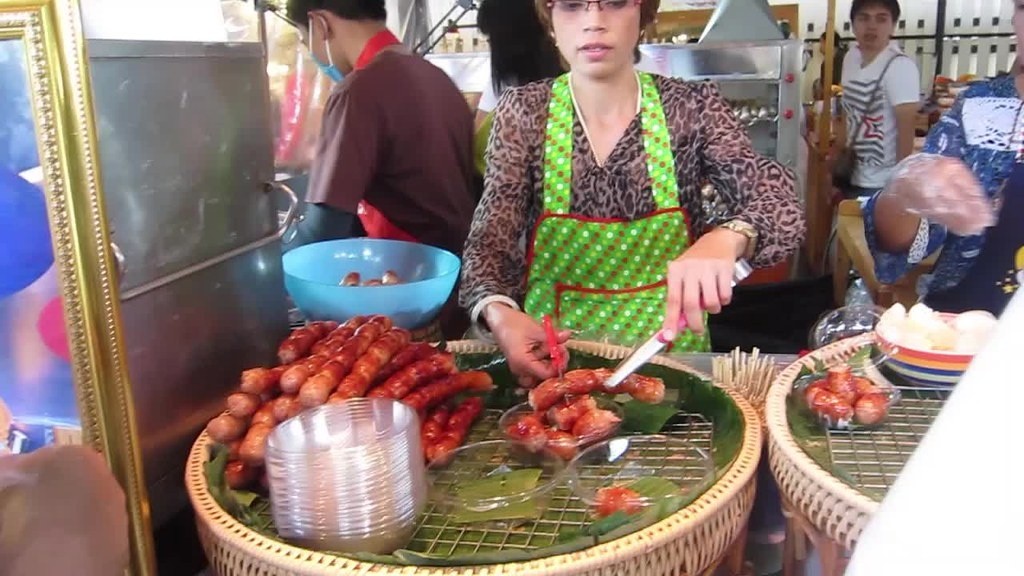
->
[[306, 15, 344, 82]]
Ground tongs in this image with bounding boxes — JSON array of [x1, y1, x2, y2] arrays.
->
[[605, 259, 755, 389]]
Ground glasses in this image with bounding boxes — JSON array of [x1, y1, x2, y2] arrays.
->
[[546, 0, 644, 13]]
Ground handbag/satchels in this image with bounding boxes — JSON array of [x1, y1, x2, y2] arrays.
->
[[830, 147, 856, 179]]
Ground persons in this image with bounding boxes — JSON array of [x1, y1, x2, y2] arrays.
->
[[275, 1, 482, 338], [845, 0, 1022, 315], [820, 0, 926, 195], [469, 1, 562, 133], [453, 1, 805, 375], [810, 32, 847, 101], [0, 441, 128, 573]]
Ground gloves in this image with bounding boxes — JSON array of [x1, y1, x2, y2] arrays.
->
[[884, 152, 997, 236]]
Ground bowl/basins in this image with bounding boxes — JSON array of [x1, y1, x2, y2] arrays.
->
[[874, 312, 974, 390], [264, 396, 426, 553], [283, 240, 461, 331], [501, 393, 623, 464], [568, 435, 715, 523], [791, 303, 901, 434], [428, 441, 568, 528]]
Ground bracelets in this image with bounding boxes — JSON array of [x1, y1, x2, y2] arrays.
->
[[469, 295, 523, 343], [715, 220, 757, 263]]
